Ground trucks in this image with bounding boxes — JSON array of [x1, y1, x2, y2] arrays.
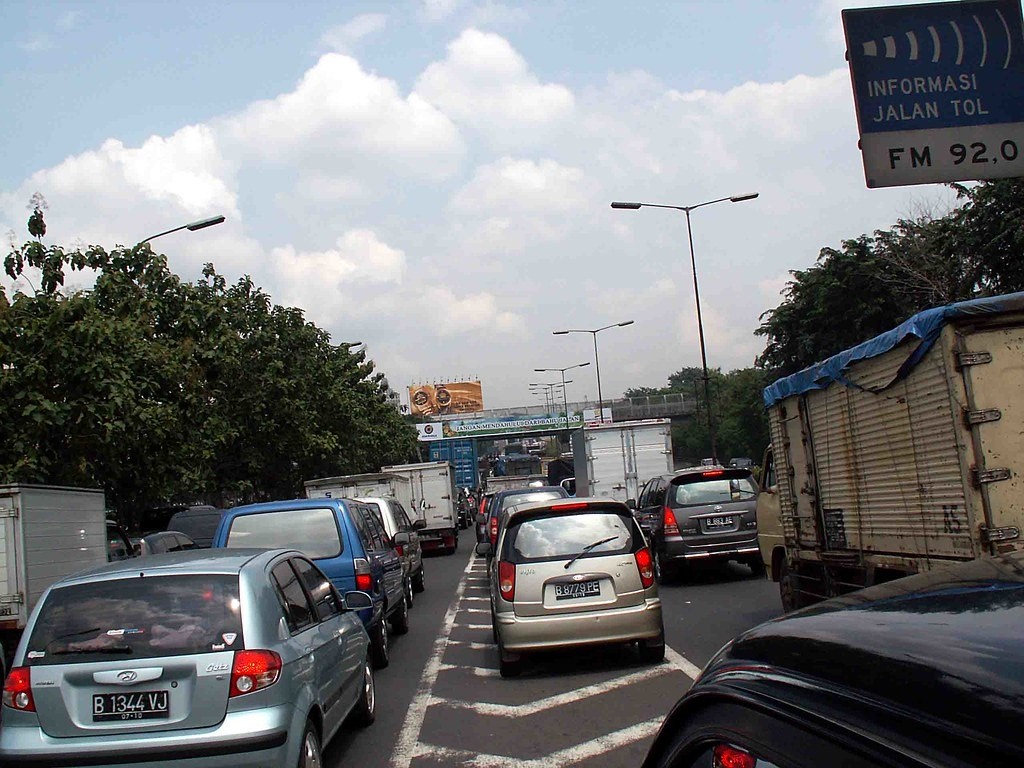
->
[[305, 473, 428, 528], [0, 483, 137, 664], [378, 459, 467, 558], [430, 437, 482, 497], [727, 290, 1024, 615], [572, 418, 675, 509]]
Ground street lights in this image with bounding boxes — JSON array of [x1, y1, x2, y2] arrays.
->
[[552, 320, 635, 422], [528, 379, 575, 413], [534, 362, 590, 429], [609, 189, 762, 466]]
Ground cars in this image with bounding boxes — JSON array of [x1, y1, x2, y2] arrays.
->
[[454, 483, 478, 525], [352, 495, 429, 608], [0, 547, 377, 768], [473, 494, 665, 675], [471, 453, 575, 578], [139, 530, 200, 556]]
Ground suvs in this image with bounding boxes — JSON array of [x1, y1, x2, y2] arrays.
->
[[635, 550, 1024, 768], [625, 461, 771, 588]]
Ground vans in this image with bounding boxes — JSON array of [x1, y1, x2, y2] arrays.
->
[[165, 508, 228, 550], [211, 496, 411, 668]]
